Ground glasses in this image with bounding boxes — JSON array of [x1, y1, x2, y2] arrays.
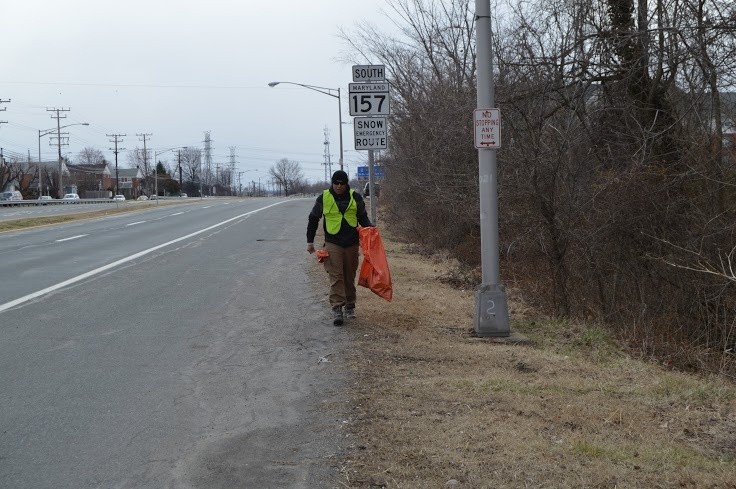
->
[[334, 181, 346, 185]]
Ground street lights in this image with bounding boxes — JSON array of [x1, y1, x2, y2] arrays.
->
[[238, 169, 258, 187], [38, 123, 89, 194], [155, 146, 187, 199], [269, 83, 344, 173]]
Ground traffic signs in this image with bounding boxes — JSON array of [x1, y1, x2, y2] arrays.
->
[[474, 108, 501, 150]]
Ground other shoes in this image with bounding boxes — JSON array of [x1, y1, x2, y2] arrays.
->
[[333, 312, 344, 325], [345, 309, 357, 320]]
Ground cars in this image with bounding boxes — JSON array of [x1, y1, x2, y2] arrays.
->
[[178, 193, 186, 198], [38, 196, 52, 205], [61, 194, 80, 204], [137, 195, 156, 201], [363, 182, 380, 198], [113, 195, 125, 201]]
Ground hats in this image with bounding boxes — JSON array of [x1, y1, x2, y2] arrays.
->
[[332, 170, 349, 183]]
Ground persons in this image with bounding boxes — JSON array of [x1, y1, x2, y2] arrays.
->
[[306, 169, 378, 326]]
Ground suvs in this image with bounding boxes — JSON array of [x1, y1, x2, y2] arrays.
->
[[0, 191, 23, 206]]
